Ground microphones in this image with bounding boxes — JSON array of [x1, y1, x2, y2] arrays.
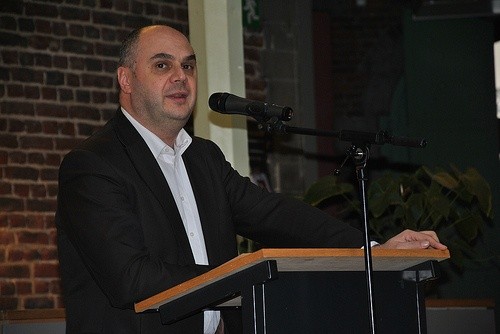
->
[[208, 92, 294, 122]]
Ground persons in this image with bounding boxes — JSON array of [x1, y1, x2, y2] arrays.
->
[[55, 24, 449, 333]]
